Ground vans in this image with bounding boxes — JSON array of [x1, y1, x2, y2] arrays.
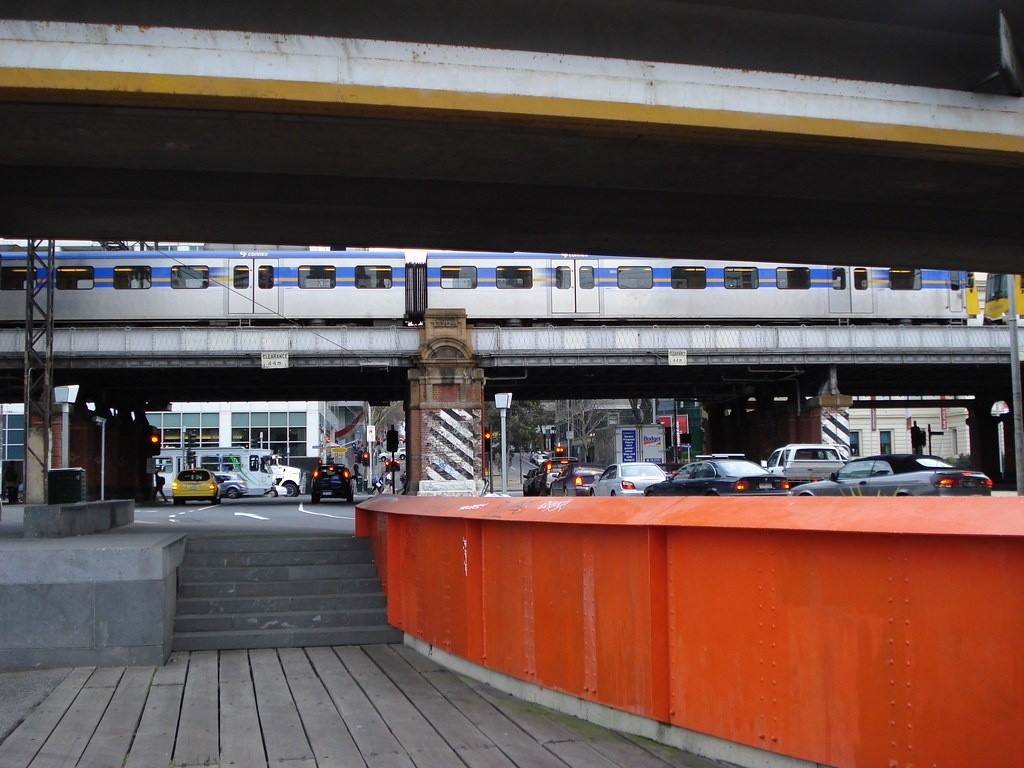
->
[[763, 443, 848, 481]]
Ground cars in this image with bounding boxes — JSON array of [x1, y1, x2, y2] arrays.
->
[[530, 449, 550, 465], [790, 454, 992, 496], [524, 457, 601, 496], [643, 458, 788, 496], [589, 462, 667, 496], [172, 469, 221, 505], [377, 446, 406, 461], [213, 475, 247, 499]]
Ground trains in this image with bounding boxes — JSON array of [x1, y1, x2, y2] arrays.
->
[[0, 248, 982, 326]]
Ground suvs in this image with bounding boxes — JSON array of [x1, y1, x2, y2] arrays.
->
[[311, 464, 353, 503]]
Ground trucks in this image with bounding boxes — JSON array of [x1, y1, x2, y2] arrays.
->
[[588, 422, 666, 467]]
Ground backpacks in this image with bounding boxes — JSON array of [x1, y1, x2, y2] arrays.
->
[[159, 477, 165, 485]]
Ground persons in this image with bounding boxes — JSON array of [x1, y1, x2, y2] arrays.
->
[[154, 471, 168, 504], [396, 471, 406, 493], [372, 472, 393, 494], [5, 461, 21, 504]]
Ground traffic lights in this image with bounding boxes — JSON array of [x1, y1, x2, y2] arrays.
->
[[911, 426, 921, 447], [188, 451, 196, 464], [362, 453, 370, 466], [149, 426, 160, 454], [485, 431, 490, 451], [387, 430, 398, 452], [188, 432, 197, 446]]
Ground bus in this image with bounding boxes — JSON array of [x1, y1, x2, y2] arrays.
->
[[152, 447, 277, 497]]
